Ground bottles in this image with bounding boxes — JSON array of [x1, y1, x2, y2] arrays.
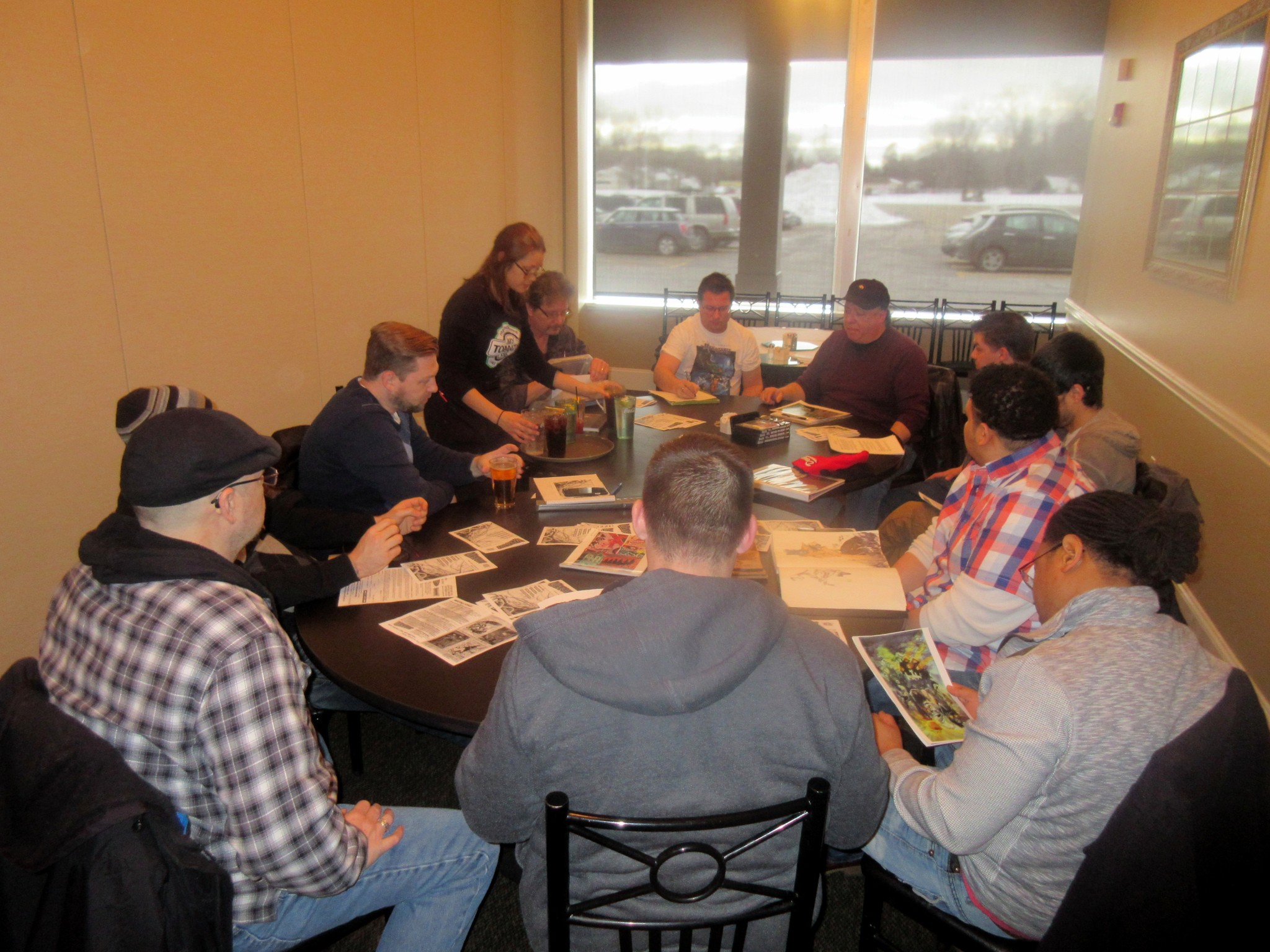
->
[[768, 343, 774, 360], [789, 332, 797, 351]]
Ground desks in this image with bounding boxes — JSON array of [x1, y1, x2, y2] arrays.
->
[[748, 326, 833, 389], [272, 388, 903, 735]]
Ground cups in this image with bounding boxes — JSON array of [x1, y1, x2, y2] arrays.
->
[[604, 385, 626, 433], [520, 397, 586, 459], [489, 456, 519, 510], [614, 394, 636, 440]]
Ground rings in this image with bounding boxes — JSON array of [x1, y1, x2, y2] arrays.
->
[[378, 818, 390, 832], [601, 368, 604, 371]]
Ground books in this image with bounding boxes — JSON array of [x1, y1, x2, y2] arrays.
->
[[770, 529, 908, 612]]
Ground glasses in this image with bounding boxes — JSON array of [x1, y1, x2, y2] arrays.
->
[[513, 260, 546, 279], [211, 467, 280, 509], [701, 302, 732, 314], [1019, 542, 1086, 590], [538, 306, 574, 322]]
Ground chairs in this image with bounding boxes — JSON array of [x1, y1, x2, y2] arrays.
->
[[261, 425, 308, 541], [863, 671, 1270, 952], [650, 287, 1066, 373], [1131, 459, 1201, 628], [0, 655, 392, 952], [546, 777, 831, 952]]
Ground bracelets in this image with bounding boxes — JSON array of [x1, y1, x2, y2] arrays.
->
[[496, 410, 504, 426]]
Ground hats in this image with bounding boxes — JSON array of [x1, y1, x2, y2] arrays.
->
[[838, 278, 890, 310], [119, 407, 282, 508], [115, 385, 213, 444]]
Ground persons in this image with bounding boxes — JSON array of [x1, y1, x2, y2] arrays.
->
[[762, 278, 930, 441], [297, 320, 525, 517], [37, 385, 501, 952], [863, 310, 1233, 942], [457, 434, 888, 952], [653, 273, 762, 401], [424, 221, 611, 457]]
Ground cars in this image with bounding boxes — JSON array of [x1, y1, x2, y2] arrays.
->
[[595, 188, 804, 257], [940, 203, 1079, 274], [1154, 188, 1239, 260]]
